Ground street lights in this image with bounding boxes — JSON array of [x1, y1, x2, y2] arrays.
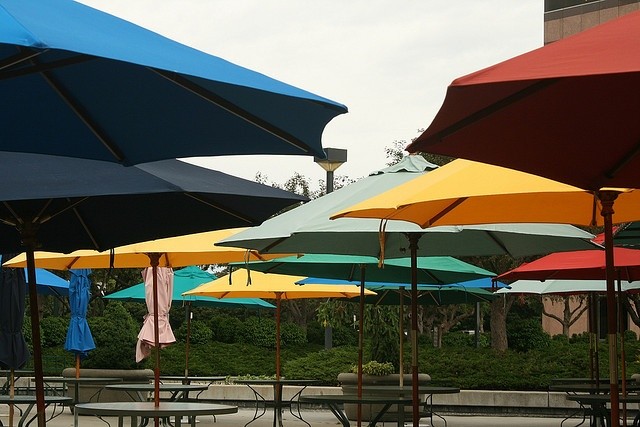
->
[[314, 148, 347, 349]]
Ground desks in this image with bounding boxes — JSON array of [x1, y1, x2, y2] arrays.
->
[[31, 377, 124, 427], [551, 376, 638, 385], [234, 379, 324, 427], [559, 395, 639, 427], [343, 385, 461, 427], [150, 374, 225, 400], [73, 401, 238, 426], [0, 395, 74, 427], [298, 393, 422, 427], [0, 369, 36, 395], [538, 384, 640, 427], [107, 385, 209, 427]]
